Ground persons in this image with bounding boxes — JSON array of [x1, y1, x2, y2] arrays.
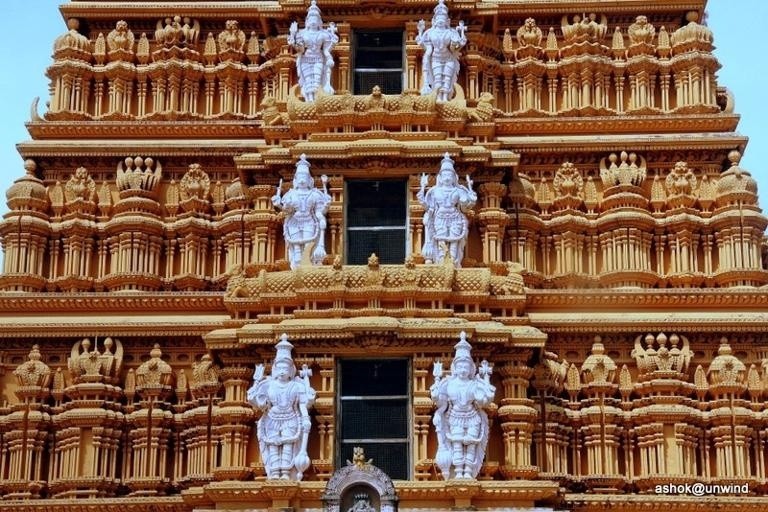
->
[[246, 330, 317, 480], [288, 3, 337, 104], [416, 2, 466, 104], [416, 154, 477, 269], [427, 332, 498, 478], [271, 154, 331, 269]]
